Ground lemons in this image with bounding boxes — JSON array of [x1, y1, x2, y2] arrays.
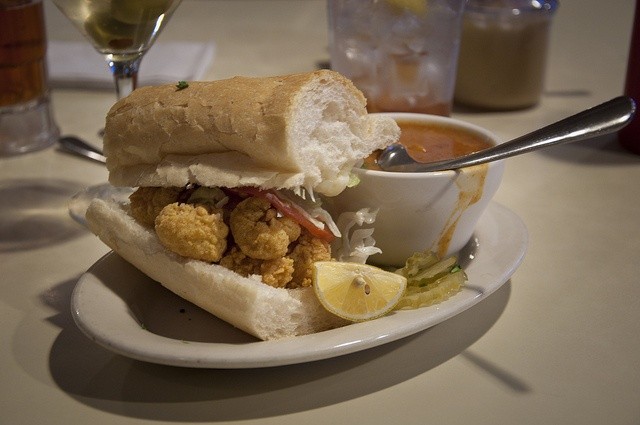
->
[[310, 261, 407, 324]]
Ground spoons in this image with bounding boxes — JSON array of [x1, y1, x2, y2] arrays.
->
[[377, 93, 637, 173]]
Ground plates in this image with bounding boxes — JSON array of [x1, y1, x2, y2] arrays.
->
[[69, 198, 529, 370]]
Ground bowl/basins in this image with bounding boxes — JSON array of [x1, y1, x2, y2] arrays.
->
[[318, 115, 506, 265]]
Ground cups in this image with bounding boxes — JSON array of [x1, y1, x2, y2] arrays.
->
[[452, 1, 559, 109], [327, 0, 464, 116]]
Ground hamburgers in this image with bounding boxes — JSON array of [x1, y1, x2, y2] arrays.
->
[[69, 67, 403, 343]]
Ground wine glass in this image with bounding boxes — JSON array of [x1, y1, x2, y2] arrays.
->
[[50, 0, 181, 223]]
